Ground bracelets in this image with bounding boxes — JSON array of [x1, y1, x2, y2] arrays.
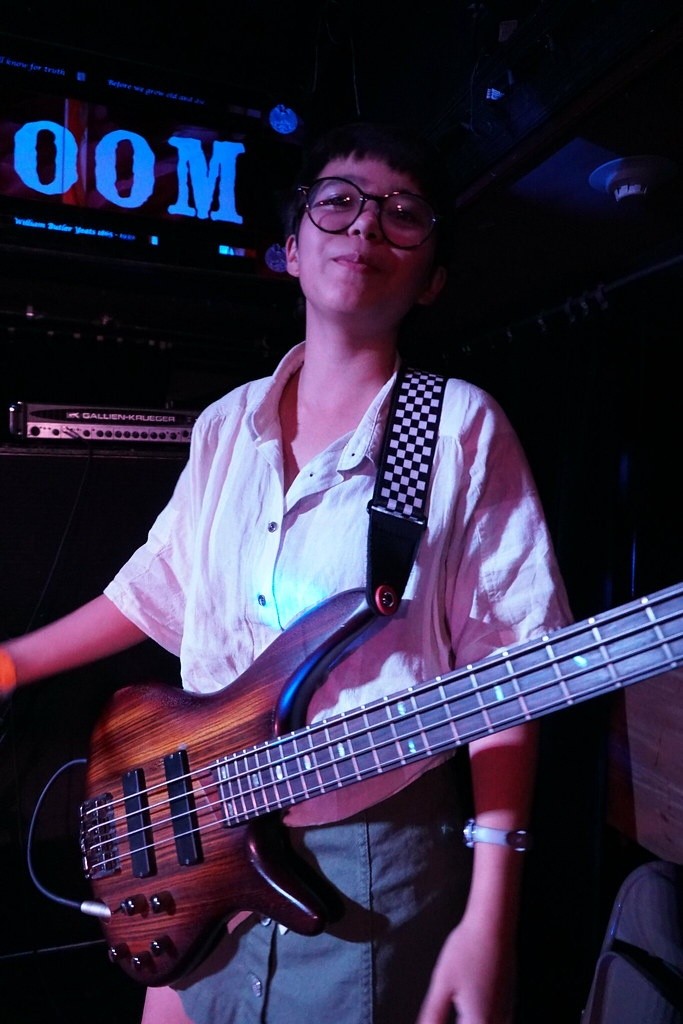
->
[[461, 813, 528, 855]]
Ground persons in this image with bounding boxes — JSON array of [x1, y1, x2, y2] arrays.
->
[[0, 127, 574, 1023]]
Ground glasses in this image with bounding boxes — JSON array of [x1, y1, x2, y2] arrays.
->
[[291, 174, 442, 250]]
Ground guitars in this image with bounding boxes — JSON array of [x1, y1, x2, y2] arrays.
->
[[78, 577, 683, 988]]
